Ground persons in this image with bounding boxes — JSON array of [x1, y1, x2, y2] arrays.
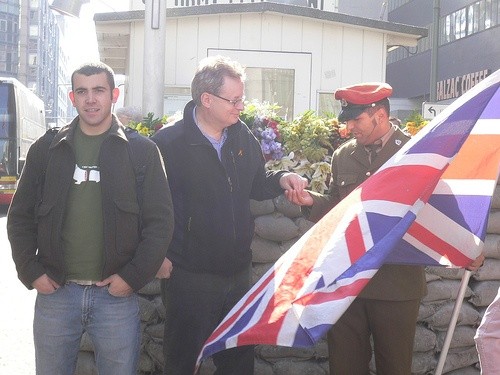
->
[[473, 287, 500, 375], [146, 58, 308, 375], [284, 83, 485, 375], [7, 61, 173, 375]]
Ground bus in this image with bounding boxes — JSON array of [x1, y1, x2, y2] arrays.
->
[[0, 77, 46, 205]]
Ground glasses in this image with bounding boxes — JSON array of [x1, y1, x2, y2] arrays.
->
[[213, 93, 247, 106]]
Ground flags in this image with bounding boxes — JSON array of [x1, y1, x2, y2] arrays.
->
[[195, 70, 500, 375]]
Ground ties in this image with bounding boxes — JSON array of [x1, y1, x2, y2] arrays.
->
[[365, 144, 381, 164]]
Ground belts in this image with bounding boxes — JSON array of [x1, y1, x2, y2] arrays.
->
[[70, 278, 99, 286]]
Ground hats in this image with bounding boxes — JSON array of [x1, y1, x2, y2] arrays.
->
[[335, 82, 392, 122]]
